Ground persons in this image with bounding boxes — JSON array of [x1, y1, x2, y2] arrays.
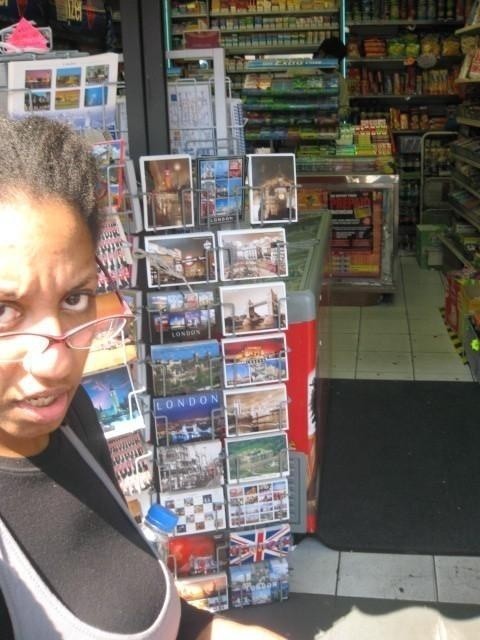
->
[[0, 114, 289, 639], [302, 35, 351, 124]]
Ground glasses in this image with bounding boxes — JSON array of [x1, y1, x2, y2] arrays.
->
[[0, 253, 135, 363]]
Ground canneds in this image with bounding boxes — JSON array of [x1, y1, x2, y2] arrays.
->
[[362, 68, 400, 95]]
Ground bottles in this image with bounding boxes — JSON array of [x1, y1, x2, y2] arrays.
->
[[389, 102, 429, 131], [397, 136, 420, 252]]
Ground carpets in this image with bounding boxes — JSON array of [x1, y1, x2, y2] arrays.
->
[[315, 378, 480, 558]]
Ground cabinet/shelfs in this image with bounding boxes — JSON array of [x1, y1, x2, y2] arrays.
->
[[284, 158, 401, 277], [345, 0, 463, 254], [439, 1, 480, 278], [168, 2, 345, 158]]
